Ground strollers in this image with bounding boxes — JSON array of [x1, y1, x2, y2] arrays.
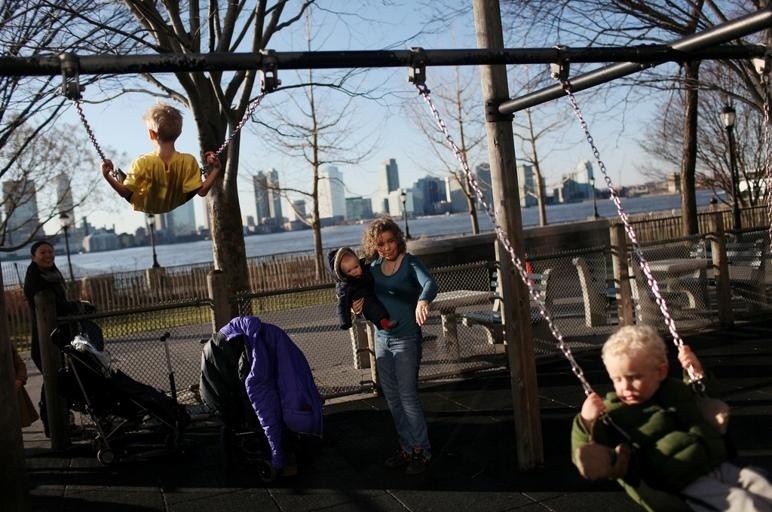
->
[[50, 325, 188, 467]]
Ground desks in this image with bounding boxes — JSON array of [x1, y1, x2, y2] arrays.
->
[[360, 288, 490, 395], [640, 258, 707, 318]]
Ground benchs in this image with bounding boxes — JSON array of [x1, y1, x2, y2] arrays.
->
[[572, 259, 636, 327], [458, 259, 559, 373], [698, 234, 770, 317]]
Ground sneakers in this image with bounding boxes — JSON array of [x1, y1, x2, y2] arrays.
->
[[405, 444, 431, 475], [386, 444, 414, 467]]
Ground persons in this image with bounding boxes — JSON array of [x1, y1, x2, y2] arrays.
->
[[23, 240, 100, 439], [349, 213, 440, 477], [10, 342, 41, 432], [326, 247, 398, 332], [101, 102, 223, 217], [570, 321, 771, 511]]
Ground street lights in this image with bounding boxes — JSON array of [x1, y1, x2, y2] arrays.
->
[[590, 176, 599, 217], [59, 212, 75, 282], [146, 213, 160, 267], [720, 105, 743, 244], [400, 191, 411, 240]]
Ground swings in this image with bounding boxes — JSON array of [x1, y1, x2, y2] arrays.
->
[[59, 49, 281, 202], [407, 45, 740, 511]]
